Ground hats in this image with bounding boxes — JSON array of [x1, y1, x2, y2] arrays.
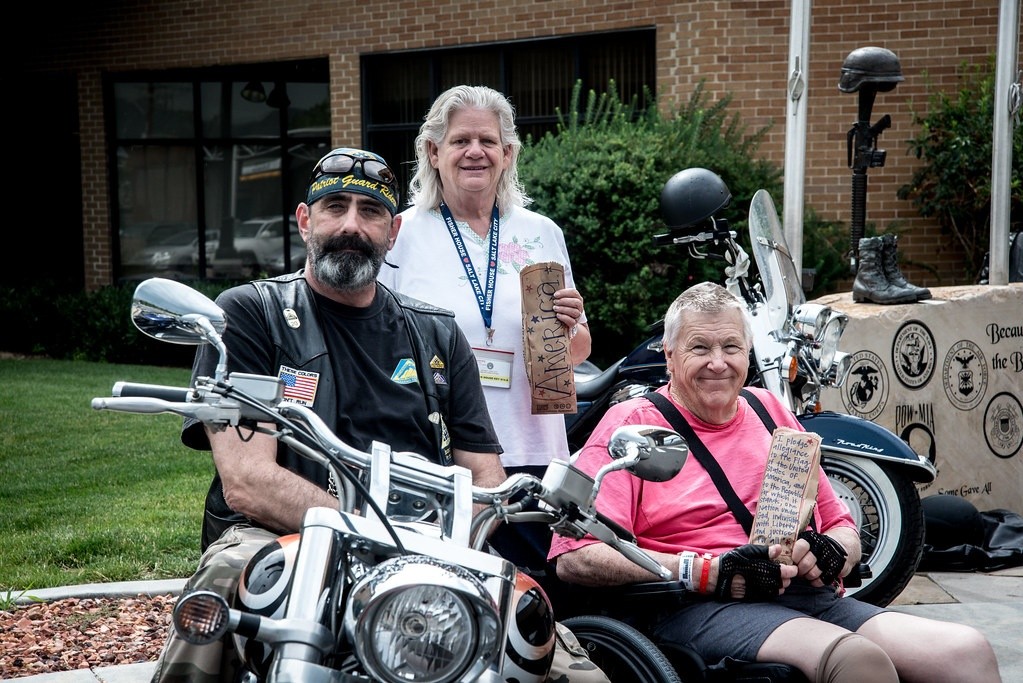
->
[[307, 148, 398, 217]]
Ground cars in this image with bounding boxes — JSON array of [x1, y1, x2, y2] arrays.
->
[[128, 229, 220, 271], [192, 216, 305, 267]]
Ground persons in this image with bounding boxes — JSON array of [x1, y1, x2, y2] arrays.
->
[[548, 285, 1002, 683], [376, 84, 592, 571], [180, 148, 508, 555]]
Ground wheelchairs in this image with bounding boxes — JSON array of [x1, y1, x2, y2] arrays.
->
[[558, 615, 813, 683]]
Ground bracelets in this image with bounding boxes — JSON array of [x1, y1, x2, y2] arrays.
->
[[679, 550, 699, 591], [698, 552, 712, 593]]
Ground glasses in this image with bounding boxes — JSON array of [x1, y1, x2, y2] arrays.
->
[[307, 153, 400, 204]]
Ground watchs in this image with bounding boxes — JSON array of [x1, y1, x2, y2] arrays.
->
[[569, 324, 577, 339]]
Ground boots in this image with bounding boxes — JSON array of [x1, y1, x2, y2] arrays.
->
[[852, 237, 916, 304], [881, 233, 931, 299]]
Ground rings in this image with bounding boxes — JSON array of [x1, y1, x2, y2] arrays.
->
[[575, 312, 582, 320]]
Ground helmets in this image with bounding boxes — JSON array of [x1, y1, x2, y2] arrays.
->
[[921, 493, 984, 550], [659, 167, 730, 230], [836, 46, 904, 92]]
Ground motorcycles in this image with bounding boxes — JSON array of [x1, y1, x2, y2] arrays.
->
[[564, 168, 937, 608], [90, 278, 689, 683]]
